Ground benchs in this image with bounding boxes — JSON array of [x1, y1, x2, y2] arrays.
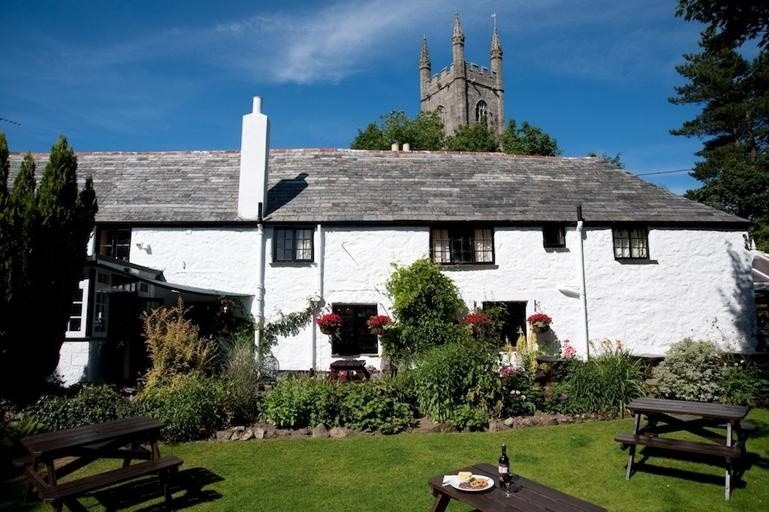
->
[[643, 415, 759, 435], [38, 454, 184, 508], [614, 434, 761, 472], [12, 439, 122, 468]]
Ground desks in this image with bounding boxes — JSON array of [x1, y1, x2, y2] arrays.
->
[[326, 360, 370, 384], [20, 416, 176, 512], [624, 396, 752, 502]]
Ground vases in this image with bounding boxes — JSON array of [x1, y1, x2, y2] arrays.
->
[[319, 326, 338, 335], [533, 325, 550, 334], [472, 326, 485, 340]]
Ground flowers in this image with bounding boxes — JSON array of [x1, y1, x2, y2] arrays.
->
[[528, 314, 552, 328], [462, 313, 493, 326], [314, 312, 344, 328], [366, 314, 392, 336]]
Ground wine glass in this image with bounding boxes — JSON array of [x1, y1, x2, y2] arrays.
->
[[502, 473, 513, 498]]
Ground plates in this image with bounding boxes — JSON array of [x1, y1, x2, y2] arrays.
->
[[448, 474, 495, 492]]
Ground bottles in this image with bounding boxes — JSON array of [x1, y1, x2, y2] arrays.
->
[[498, 443, 511, 488]]
